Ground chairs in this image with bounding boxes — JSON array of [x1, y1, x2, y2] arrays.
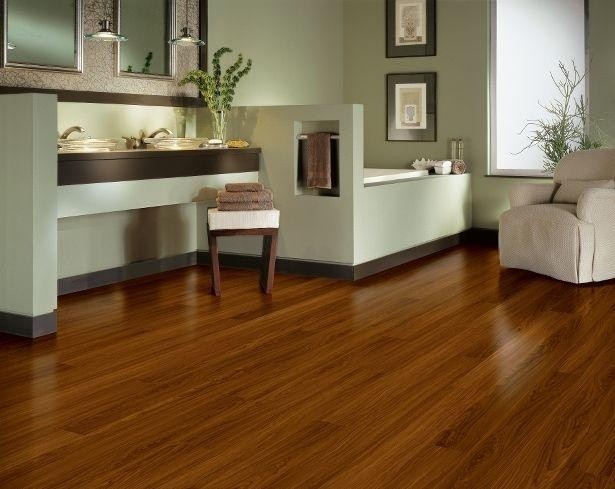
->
[[498, 149, 615, 284]]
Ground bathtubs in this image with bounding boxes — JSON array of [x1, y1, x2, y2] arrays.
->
[[364, 166, 429, 185]]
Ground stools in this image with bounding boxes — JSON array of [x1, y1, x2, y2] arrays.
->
[[207, 207, 280, 296]]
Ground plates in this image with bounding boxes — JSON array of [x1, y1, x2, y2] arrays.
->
[[142, 137, 209, 149], [58, 137, 122, 150]]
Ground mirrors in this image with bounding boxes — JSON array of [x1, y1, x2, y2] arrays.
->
[[0, 0, 83, 74], [113, 0, 177, 81]]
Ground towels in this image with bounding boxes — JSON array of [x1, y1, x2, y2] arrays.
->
[[225, 183, 264, 191], [216, 191, 273, 202], [208, 208, 279, 230], [307, 132, 339, 197], [217, 202, 273, 211]]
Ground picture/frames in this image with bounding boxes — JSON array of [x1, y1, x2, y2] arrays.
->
[[386, 0, 436, 58], [386, 72, 437, 142]]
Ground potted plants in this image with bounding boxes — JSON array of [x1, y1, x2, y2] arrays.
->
[[178, 47, 252, 144]]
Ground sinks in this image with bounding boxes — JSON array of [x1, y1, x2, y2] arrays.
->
[[147, 138, 209, 150], [59, 139, 118, 153]]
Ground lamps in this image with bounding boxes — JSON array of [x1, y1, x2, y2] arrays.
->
[[83, 0, 129, 41], [167, 0, 205, 46]]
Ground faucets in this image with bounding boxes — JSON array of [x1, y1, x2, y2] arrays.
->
[[60, 124, 87, 139], [139, 126, 174, 143]]
